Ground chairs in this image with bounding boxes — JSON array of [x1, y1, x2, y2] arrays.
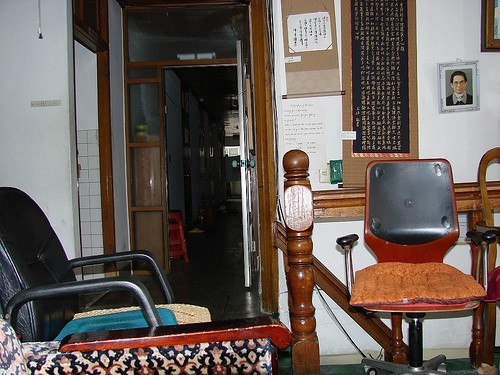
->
[[0, 186, 211, 342], [476, 148, 500, 367], [336, 157, 497, 375]]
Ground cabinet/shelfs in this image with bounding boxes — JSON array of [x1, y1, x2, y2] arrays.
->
[[127, 66, 171, 276]]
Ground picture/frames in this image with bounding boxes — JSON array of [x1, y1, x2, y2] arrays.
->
[[436, 60, 480, 114], [480, 0, 500, 52]]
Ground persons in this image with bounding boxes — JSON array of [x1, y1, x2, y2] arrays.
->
[[446, 71, 473, 106]]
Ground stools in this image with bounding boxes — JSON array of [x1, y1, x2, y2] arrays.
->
[[169, 209, 190, 265]]
